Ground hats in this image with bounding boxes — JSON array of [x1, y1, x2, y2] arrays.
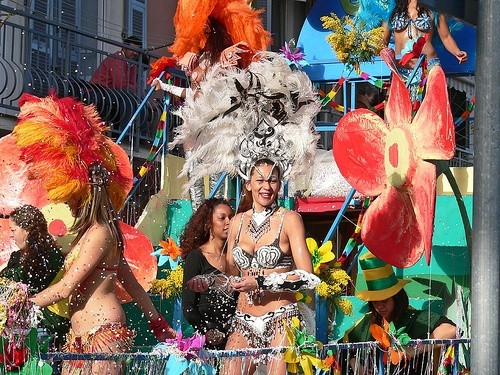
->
[[355, 252, 412, 301]]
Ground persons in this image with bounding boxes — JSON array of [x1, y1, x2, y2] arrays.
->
[[187, 158, 312, 375], [377, 0, 467, 122], [150, 19, 244, 213], [0, 205, 63, 308], [328, 253, 463, 375], [27, 161, 181, 375], [92, 35, 179, 103], [181, 197, 246, 354]]
